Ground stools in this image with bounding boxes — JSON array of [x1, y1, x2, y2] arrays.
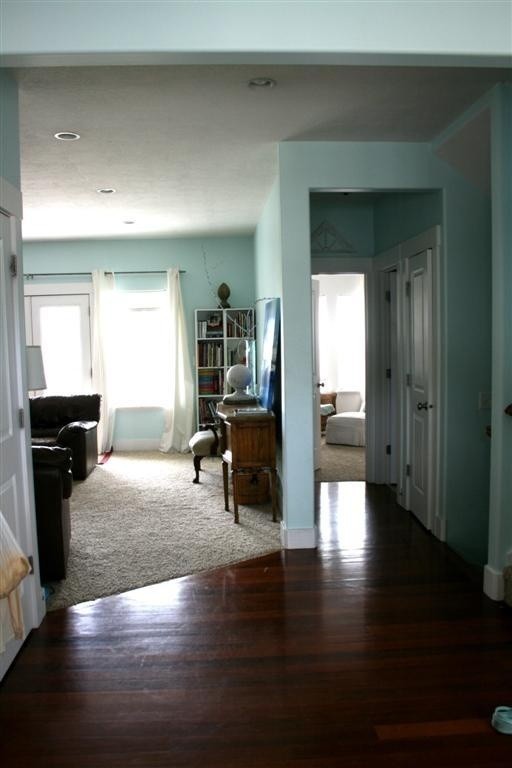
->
[[322, 409, 366, 448]]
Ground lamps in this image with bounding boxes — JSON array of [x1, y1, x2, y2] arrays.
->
[[24, 343, 47, 397]]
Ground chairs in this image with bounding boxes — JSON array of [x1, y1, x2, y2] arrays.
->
[[187, 421, 221, 486], [318, 392, 338, 437]]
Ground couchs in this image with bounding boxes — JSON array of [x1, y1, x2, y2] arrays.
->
[[26, 390, 106, 482], [27, 444, 75, 586]]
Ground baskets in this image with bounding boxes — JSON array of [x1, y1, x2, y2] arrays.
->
[[233, 469, 270, 505]]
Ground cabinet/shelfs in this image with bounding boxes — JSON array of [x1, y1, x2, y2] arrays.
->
[[193, 305, 260, 432], [212, 400, 282, 526]]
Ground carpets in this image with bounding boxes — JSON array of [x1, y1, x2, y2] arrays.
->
[[42, 448, 282, 614], [319, 425, 371, 486]]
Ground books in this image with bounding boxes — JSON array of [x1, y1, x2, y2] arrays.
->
[[197, 313, 252, 429]]
